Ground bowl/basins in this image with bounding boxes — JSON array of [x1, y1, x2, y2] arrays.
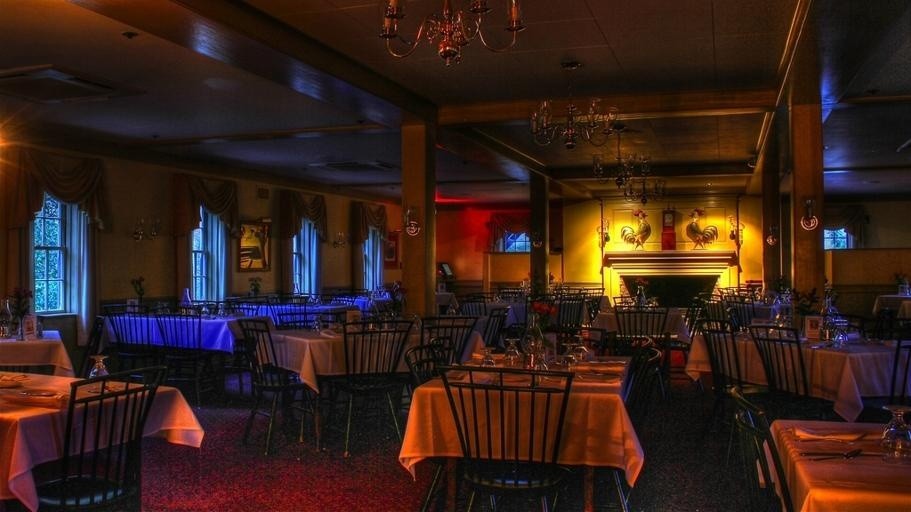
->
[[799, 338, 808, 344]]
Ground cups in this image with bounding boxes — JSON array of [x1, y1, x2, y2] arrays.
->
[[180, 308, 185, 316], [884, 337, 893, 349], [188, 308, 193, 316], [865, 332, 874, 342]]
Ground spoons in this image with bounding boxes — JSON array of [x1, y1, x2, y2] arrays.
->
[[799, 449, 862, 458]]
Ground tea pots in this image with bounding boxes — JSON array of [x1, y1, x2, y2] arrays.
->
[[193, 302, 211, 316], [377, 311, 398, 331], [817, 326, 840, 347], [832, 331, 848, 345]]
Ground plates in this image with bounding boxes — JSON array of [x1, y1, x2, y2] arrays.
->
[[579, 374, 623, 382], [1, 389, 65, 404], [808, 345, 823, 350], [589, 361, 628, 365]]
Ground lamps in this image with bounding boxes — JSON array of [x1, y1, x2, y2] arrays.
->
[[801, 197, 819, 230], [127, 217, 161, 243], [333, 231, 346, 250], [530, 60, 618, 148], [767, 225, 779, 245], [623, 177, 665, 206], [532, 230, 543, 249], [405, 206, 421, 236], [379, 1, 527, 68], [591, 123, 652, 188]]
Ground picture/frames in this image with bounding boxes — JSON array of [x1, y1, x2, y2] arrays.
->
[[237, 220, 272, 272], [384, 230, 400, 269]]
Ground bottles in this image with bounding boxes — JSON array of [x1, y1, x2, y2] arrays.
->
[[635, 284, 646, 310], [368, 294, 377, 330]]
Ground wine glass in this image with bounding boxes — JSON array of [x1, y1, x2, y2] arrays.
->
[[480, 347, 496, 368], [562, 343, 578, 381], [532, 350, 548, 385], [218, 303, 226, 319], [503, 338, 521, 368], [574, 333, 588, 367], [89, 355, 111, 393], [881, 406, 910, 467], [774, 315, 785, 345], [311, 314, 321, 336], [447, 309, 456, 326]]
